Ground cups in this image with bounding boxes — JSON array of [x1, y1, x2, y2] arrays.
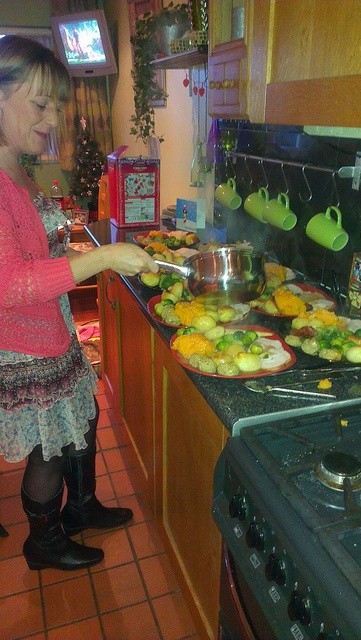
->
[[244, 187, 269, 224], [263, 192, 297, 231], [305, 206, 349, 251], [215, 178, 242, 210]]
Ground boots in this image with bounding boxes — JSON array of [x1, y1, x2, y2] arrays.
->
[[20, 481, 103, 570], [59, 444, 133, 536]]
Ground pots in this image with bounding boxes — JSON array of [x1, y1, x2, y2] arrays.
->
[[153, 248, 267, 307]]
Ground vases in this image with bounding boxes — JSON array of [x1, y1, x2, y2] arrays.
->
[[68, 129, 105, 221]]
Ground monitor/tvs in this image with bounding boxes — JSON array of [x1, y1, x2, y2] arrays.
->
[[47, 10, 118, 79]]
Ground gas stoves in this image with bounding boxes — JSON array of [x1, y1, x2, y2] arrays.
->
[[211, 404, 361, 639]]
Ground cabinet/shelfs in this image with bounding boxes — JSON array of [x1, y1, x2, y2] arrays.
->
[[83, 222, 229, 640], [209, 0, 361, 127]]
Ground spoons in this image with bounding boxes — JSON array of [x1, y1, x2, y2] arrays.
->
[[262, 394, 333, 403], [241, 380, 338, 398]]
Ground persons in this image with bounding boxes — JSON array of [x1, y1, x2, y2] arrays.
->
[[0, 35, 160, 572]]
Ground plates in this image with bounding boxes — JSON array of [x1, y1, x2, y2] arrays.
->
[[251, 282, 341, 318], [147, 294, 250, 328], [170, 324, 297, 378], [137, 274, 160, 289], [286, 321, 360, 370], [132, 230, 200, 248]]
